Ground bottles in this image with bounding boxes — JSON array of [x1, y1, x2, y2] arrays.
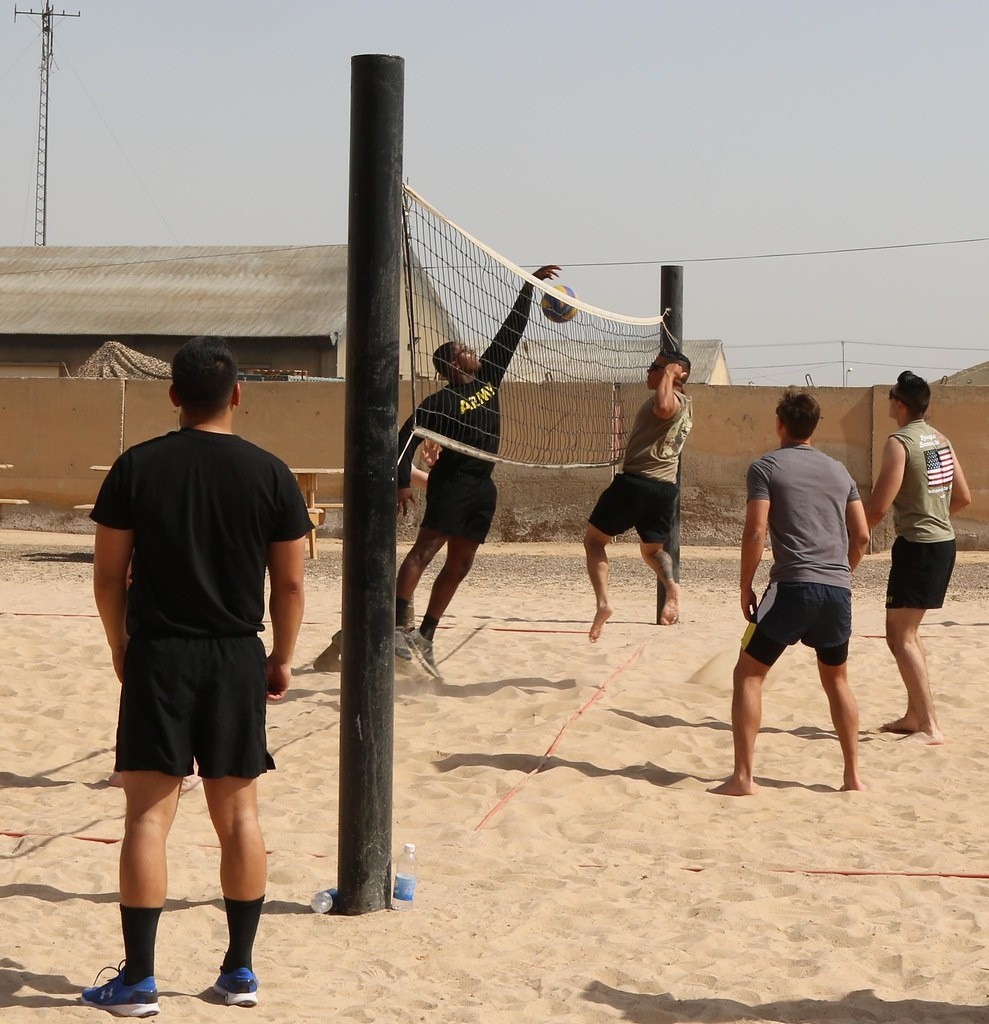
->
[[311, 886, 338, 913], [392, 844, 416, 910]]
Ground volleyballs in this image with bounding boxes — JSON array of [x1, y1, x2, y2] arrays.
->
[[540, 284, 579, 324]]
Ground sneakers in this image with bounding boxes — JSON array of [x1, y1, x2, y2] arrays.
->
[[81, 959, 160, 1017], [213, 966, 259, 1007], [404, 628, 440, 678], [396, 631, 412, 661]]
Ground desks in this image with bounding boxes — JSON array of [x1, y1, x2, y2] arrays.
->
[[74, 466, 345, 558]]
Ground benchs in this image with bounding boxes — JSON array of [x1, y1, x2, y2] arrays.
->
[[69, 504, 322, 532], [305, 501, 344, 527]]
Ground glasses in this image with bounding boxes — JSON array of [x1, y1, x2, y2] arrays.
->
[[650, 362, 664, 371], [453, 346, 469, 360], [889, 389, 909, 406]]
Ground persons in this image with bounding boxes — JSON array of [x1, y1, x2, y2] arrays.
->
[[396, 265, 566, 679], [582, 349, 694, 641], [866, 371, 972, 744], [82, 337, 305, 1019], [705, 386, 872, 797]]
